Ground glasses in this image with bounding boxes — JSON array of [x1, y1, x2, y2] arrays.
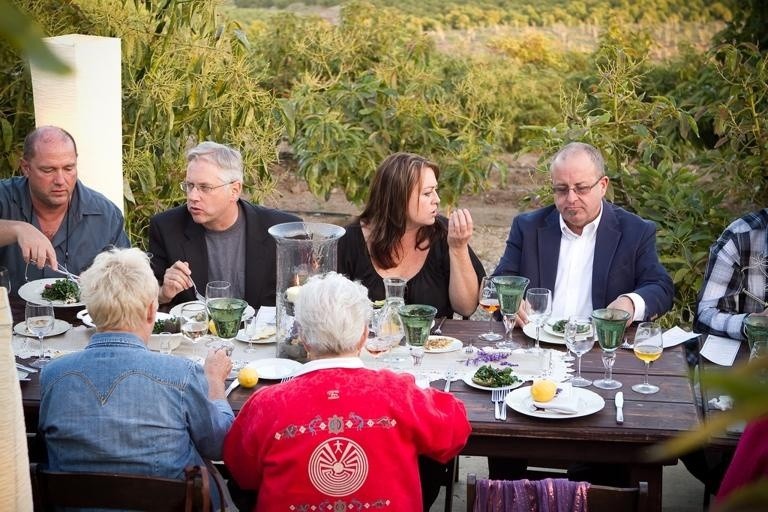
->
[[178, 180, 236, 196], [549, 178, 603, 196]]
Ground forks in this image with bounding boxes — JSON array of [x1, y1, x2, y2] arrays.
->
[[491, 390, 501, 420], [500, 389, 510, 420], [281, 377, 292, 383]]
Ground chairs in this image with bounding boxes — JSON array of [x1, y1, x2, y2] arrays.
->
[[462, 471, 649, 512], [27, 460, 211, 510], [436, 298, 470, 510]]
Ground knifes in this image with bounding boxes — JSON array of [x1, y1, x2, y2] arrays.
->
[[224, 377, 241, 398], [443, 363, 456, 393], [614, 391, 624, 423]]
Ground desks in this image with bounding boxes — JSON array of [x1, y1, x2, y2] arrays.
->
[[697, 333, 768, 512], [8, 289, 703, 511]]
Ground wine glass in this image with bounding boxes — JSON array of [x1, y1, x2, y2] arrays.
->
[[562, 316, 593, 387], [524, 288, 552, 354], [494, 277, 530, 349], [15, 277, 437, 376], [477, 277, 504, 342], [631, 320, 664, 394], [590, 309, 629, 390]]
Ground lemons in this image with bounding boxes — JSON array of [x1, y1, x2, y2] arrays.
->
[[531, 375, 560, 403], [236, 366, 262, 390], [207, 319, 221, 337]]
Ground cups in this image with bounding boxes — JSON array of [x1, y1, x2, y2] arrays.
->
[[269, 222, 347, 364], [0, 267, 11, 296], [743, 314, 767, 355]]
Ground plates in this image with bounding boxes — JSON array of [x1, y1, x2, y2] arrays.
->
[[426, 334, 464, 354], [543, 317, 591, 337], [506, 382, 606, 421], [460, 363, 528, 391]]
